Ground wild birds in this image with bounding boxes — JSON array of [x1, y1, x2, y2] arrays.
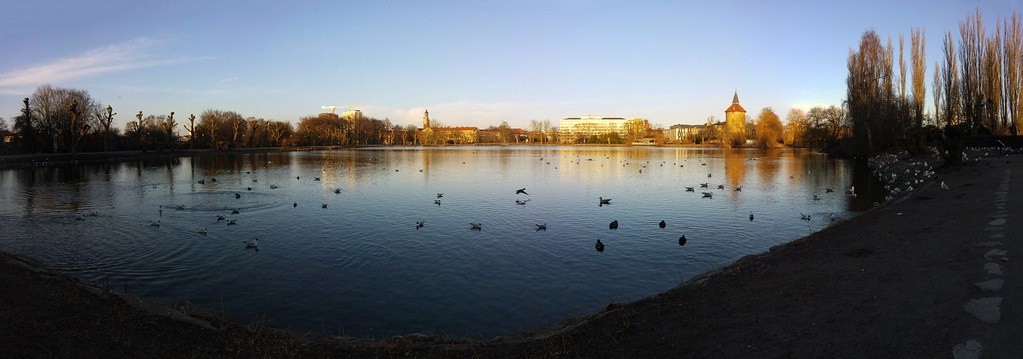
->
[[659, 220, 666, 228], [536, 223, 546, 230], [749, 211, 754, 221], [416, 220, 424, 227], [843, 144, 1023, 201], [678, 235, 686, 246], [800, 212, 811, 219], [825, 187, 833, 192], [609, 220, 618, 229], [516, 188, 529, 195], [598, 197, 611, 204], [594, 239, 604, 252], [830, 212, 841, 220], [433, 199, 441, 205], [436, 192, 443, 197], [241, 236, 260, 248], [198, 227, 209, 236], [469, 222, 482, 229], [812, 194, 821, 200], [685, 173, 742, 199], [147, 170, 343, 227]]
